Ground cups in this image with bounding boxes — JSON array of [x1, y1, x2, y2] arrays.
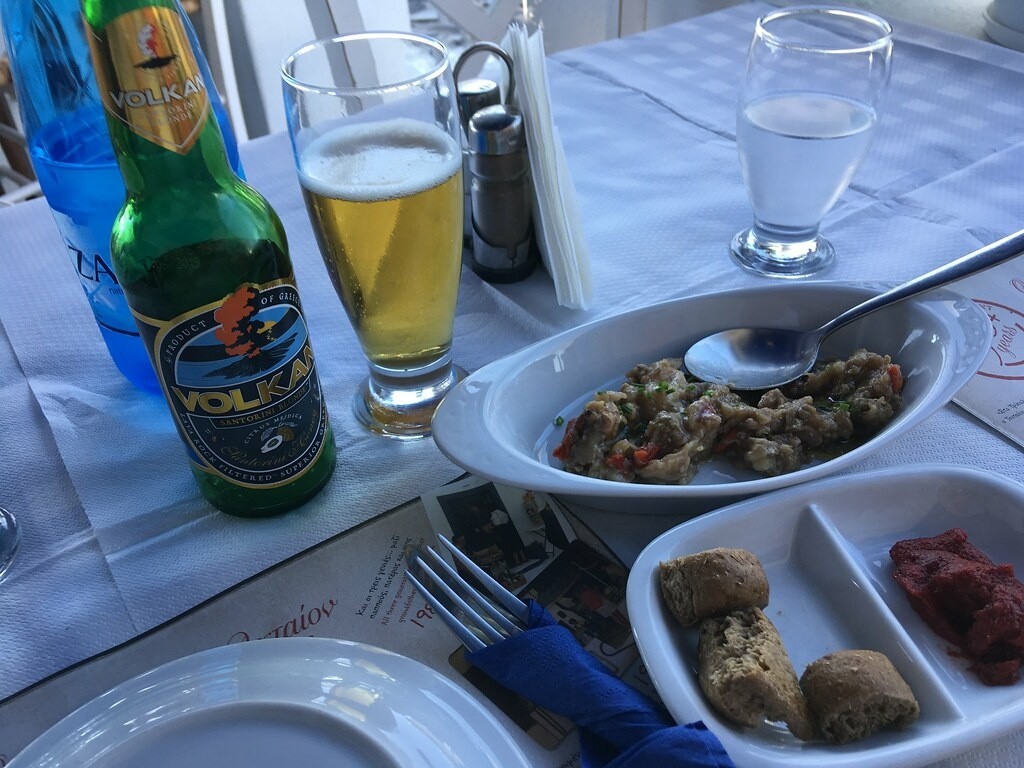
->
[[731, 6, 893, 279], [279, 30, 469, 441]]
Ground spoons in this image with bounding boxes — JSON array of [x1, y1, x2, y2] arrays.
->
[[684, 232, 1024, 392]]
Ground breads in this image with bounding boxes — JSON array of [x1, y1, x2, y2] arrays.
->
[[659, 546, 920, 744]]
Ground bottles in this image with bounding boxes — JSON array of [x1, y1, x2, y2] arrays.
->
[[80, 1, 339, 520], [469, 104, 532, 282], [2, 0, 247, 391]]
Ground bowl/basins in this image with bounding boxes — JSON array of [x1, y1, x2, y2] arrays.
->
[[432, 280, 995, 514]]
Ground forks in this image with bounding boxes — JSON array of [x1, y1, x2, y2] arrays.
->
[[404, 533, 534, 656]]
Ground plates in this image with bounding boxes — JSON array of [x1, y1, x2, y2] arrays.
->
[[624, 459, 1024, 768], [2, 638, 534, 768]]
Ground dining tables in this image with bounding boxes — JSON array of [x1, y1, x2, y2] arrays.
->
[[0, 0, 1024, 768]]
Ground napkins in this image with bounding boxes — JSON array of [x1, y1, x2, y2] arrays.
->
[[465, 596, 736, 768]]
